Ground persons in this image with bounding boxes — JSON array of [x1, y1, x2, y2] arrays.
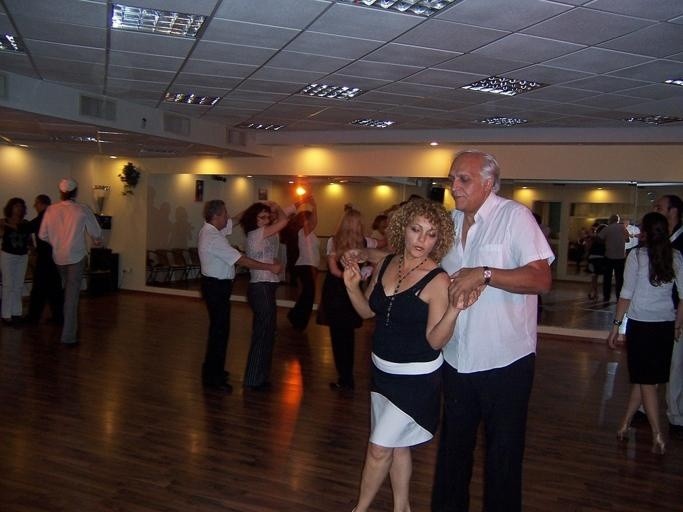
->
[[340, 150, 555, 512], [0, 196, 36, 327], [199, 196, 321, 389], [38, 176, 106, 347], [3, 193, 66, 326], [608, 195, 682, 453], [587, 216, 640, 302], [344, 200, 482, 512], [531, 214, 551, 313], [317, 192, 425, 389]]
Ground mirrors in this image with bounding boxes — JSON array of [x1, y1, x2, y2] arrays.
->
[[144, 171, 682, 337]]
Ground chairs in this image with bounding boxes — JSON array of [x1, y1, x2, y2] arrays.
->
[[0, 247, 111, 300]]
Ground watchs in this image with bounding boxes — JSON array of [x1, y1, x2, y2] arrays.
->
[[483, 266, 491, 285]]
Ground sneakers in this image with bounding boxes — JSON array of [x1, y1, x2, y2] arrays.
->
[[201, 363, 355, 394]]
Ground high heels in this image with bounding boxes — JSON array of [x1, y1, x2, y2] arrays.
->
[[615, 414, 632, 442], [649, 430, 669, 458]]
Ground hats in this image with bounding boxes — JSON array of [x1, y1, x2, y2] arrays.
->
[[58, 176, 78, 193]]
